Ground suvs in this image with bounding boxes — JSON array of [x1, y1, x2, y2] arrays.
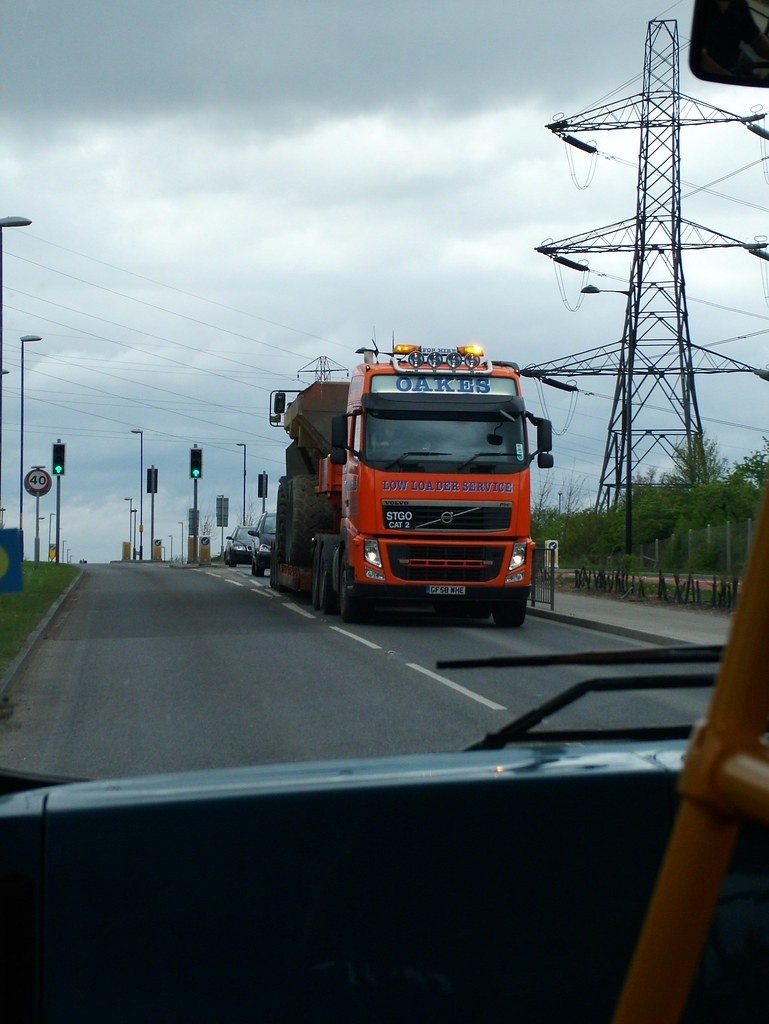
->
[[248, 510, 276, 577]]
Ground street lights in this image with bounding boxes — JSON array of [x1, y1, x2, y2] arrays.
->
[[580, 284, 634, 554], [160, 546, 166, 563], [61, 539, 67, 563], [168, 535, 172, 562], [0, 216, 34, 506], [237, 444, 247, 526], [131, 509, 137, 560], [124, 498, 131, 541], [18, 334, 42, 530], [71, 555, 74, 564], [130, 428, 143, 561], [48, 512, 56, 563], [178, 521, 184, 563], [67, 548, 71, 563]]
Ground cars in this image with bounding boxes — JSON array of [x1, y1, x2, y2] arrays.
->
[[223, 525, 258, 569]]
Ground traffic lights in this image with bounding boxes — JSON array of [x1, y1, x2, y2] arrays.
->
[[52, 443, 65, 476], [191, 449, 202, 479]]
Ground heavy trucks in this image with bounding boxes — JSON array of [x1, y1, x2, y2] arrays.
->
[[269, 345, 554, 626]]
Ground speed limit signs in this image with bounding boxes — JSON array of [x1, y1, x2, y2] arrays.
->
[[25, 469, 50, 493]]
